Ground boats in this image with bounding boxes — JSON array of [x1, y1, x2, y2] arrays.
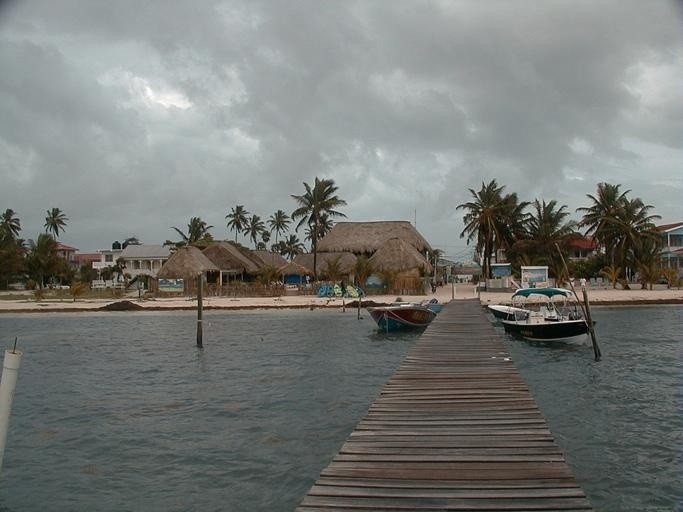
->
[[364, 305, 438, 331], [319, 284, 365, 297], [487, 288, 596, 347]]
